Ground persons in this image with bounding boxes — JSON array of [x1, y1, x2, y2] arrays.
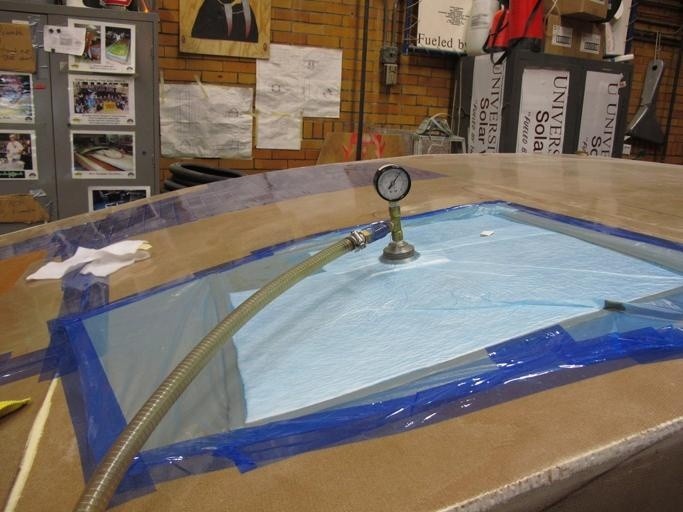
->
[[73, 80, 127, 112], [5, 133, 24, 164]]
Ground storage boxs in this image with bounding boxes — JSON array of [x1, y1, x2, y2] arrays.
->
[[576, 22, 606, 62], [543, 0, 607, 21], [543, 15, 575, 56]]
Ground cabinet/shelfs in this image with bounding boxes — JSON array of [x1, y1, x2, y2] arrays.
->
[[0, 0, 158, 235]]
